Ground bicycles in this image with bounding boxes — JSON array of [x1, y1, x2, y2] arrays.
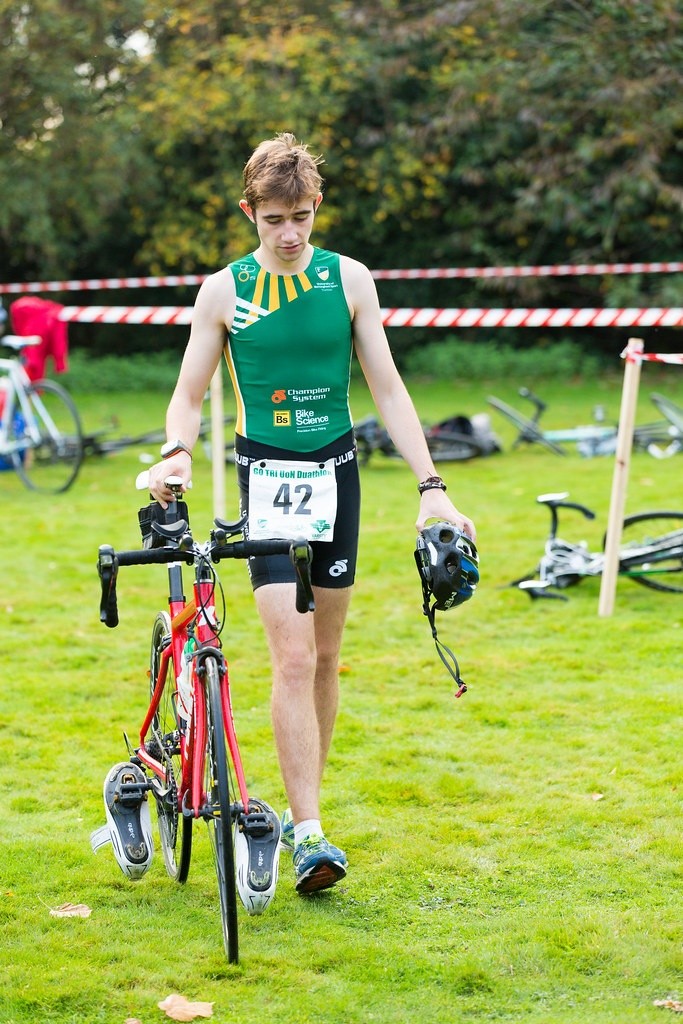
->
[[346, 411, 503, 465], [96, 477, 325, 970], [510, 489, 682, 605], [483, 382, 683, 473], [35, 411, 238, 474], [0, 302, 88, 499]]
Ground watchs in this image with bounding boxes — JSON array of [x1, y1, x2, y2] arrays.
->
[[160, 439, 193, 459]]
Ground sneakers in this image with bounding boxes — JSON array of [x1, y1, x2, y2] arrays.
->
[[294, 835, 346, 892], [234, 798, 281, 915], [278, 807, 350, 868], [103, 763, 155, 879]]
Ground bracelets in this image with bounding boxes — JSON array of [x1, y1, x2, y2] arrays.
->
[[418, 471, 446, 494]]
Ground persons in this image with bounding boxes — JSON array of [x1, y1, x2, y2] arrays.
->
[[141, 128, 475, 893]]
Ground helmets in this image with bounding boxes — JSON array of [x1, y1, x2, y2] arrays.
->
[[417, 521, 480, 610]]
